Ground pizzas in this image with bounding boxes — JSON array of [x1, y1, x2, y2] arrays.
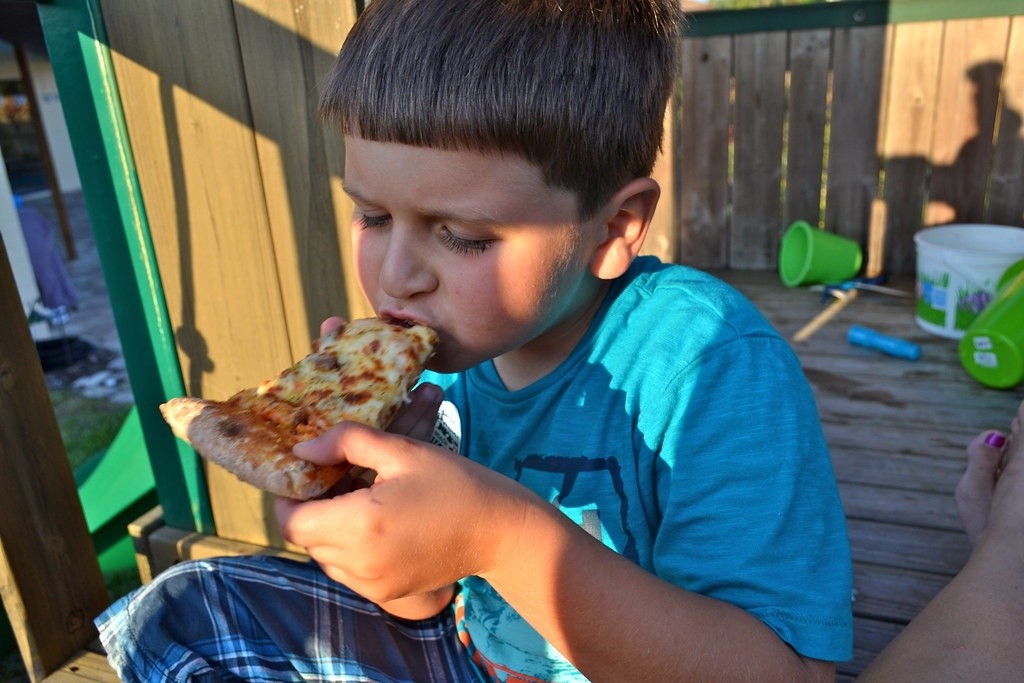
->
[[157, 315, 435, 502]]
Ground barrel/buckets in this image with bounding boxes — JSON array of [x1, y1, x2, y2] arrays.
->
[[913, 223, 1024, 342], [957, 256, 1024, 389], [777, 220, 863, 289]]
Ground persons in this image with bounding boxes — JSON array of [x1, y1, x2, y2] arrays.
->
[[856, 395, 1024, 682], [95, 1, 856, 682]]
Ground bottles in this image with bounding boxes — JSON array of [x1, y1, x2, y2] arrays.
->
[[845, 322, 922, 362]]
